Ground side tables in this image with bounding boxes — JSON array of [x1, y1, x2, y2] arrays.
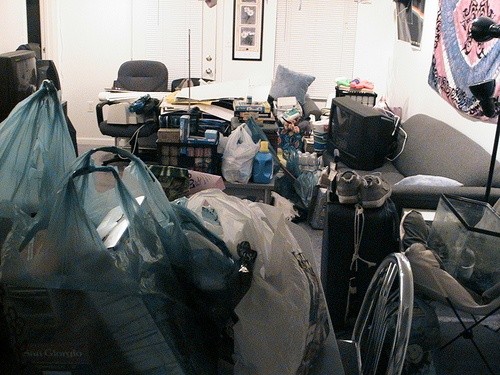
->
[[336, 86, 378, 108]]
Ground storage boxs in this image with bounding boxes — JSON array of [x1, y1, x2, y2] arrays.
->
[[427, 193, 500, 296], [273, 97, 302, 117], [8, 196, 204, 375], [302, 137, 315, 153], [158, 115, 218, 172]]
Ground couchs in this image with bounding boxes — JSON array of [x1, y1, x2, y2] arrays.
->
[[324, 113, 500, 229], [232, 98, 321, 152]]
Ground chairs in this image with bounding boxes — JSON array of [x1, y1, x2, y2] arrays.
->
[[400, 195, 500, 375], [334, 253, 414, 375], [96, 61, 169, 166], [172, 79, 214, 91], [147, 164, 226, 201]]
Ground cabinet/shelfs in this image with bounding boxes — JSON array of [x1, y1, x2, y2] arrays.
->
[[156, 122, 232, 175]]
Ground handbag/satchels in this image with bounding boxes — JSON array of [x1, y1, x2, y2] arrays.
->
[[274, 150, 307, 210], [169, 204, 257, 375], [186, 190, 311, 375], [0, 80, 77, 218], [0, 146, 217, 375], [221, 123, 261, 185]]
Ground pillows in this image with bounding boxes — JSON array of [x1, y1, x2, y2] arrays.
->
[[269, 64, 316, 102], [394, 174, 464, 188]]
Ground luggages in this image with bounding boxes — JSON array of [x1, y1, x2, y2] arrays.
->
[[322, 189, 399, 329]]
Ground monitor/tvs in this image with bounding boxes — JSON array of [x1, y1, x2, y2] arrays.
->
[[0, 49, 41, 121], [326, 95, 393, 171]]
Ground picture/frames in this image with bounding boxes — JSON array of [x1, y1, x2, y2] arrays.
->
[[231, 0, 264, 61]]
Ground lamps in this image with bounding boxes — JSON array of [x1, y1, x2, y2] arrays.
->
[[467, 16, 500, 202]]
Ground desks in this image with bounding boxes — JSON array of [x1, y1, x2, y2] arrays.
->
[[219, 176, 275, 205]]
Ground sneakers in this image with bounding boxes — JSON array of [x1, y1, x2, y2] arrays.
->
[[336, 170, 362, 204], [360, 172, 392, 209]]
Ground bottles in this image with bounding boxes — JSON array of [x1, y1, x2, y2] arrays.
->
[[253, 141, 274, 184], [288, 150, 317, 172]]
